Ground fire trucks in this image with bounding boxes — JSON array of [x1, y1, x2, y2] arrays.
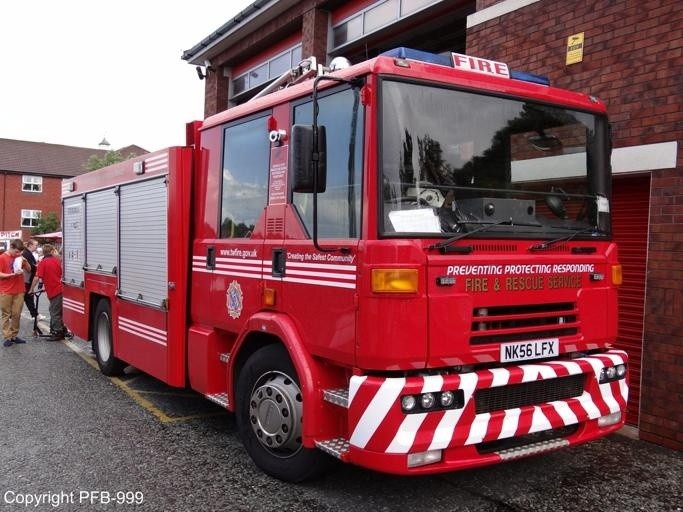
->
[[61, 44, 631, 483]]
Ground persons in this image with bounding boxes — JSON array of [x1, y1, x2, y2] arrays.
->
[[0, 238, 30, 347], [20, 237, 39, 318], [28, 243, 65, 342]]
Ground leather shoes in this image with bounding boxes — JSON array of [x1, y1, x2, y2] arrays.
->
[[11, 336, 26, 344], [44, 335, 65, 342], [2, 339, 13, 347]]
